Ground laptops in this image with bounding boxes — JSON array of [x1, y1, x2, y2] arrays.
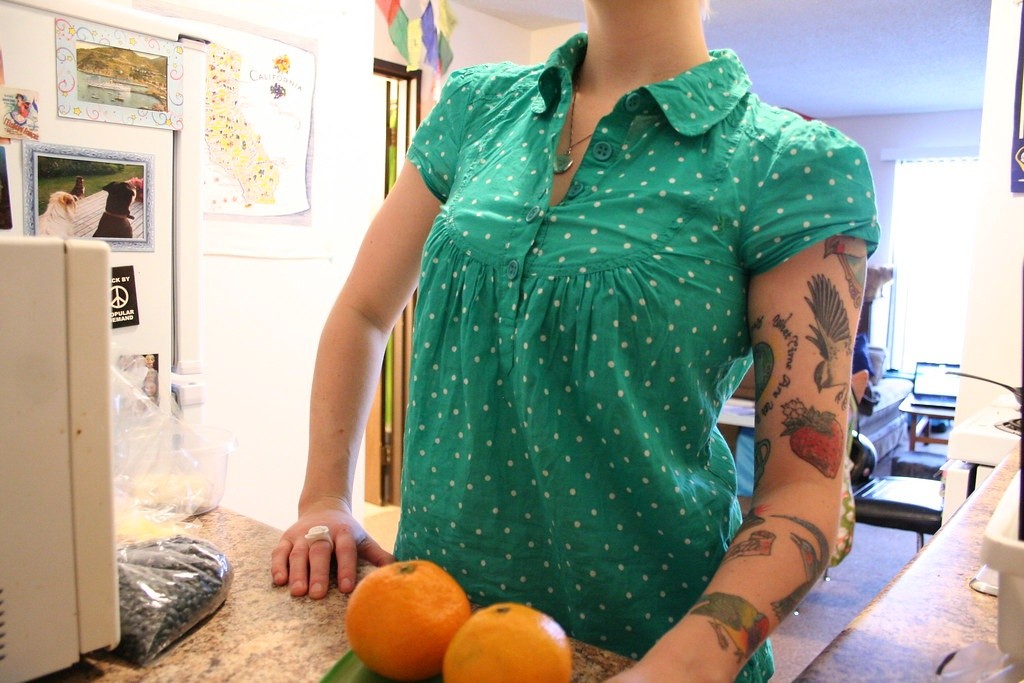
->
[[912, 362, 961, 411]]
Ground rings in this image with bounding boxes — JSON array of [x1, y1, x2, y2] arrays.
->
[[306, 526, 335, 550]]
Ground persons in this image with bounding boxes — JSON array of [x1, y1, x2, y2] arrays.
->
[[275, 0, 879, 683]]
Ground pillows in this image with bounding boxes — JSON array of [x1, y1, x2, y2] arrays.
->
[[869, 344, 887, 384]]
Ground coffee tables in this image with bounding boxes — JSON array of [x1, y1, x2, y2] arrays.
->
[[899, 394, 954, 451]]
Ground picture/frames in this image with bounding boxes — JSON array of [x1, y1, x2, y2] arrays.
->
[[21, 140, 156, 253], [54, 17, 184, 132]]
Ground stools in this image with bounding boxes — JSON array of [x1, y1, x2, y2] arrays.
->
[[855, 473, 941, 551]]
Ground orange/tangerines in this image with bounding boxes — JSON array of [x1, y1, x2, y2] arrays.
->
[[345, 560, 471, 682], [443, 603, 572, 683]]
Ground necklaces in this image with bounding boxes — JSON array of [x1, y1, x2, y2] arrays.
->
[[554, 96, 597, 174]]
